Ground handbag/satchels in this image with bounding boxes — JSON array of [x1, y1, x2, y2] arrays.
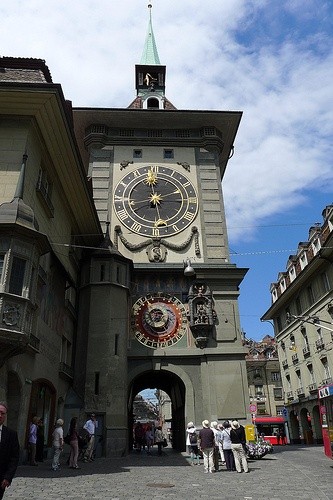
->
[[162, 438, 168, 448], [242, 441, 247, 451]]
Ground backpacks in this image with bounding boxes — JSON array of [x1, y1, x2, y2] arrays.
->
[[189, 432, 197, 444]]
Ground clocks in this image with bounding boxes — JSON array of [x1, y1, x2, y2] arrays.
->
[[113, 166, 199, 238]]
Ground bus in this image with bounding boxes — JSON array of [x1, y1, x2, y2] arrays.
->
[[132, 419, 160, 446], [251, 415, 290, 447]]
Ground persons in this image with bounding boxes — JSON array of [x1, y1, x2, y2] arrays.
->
[[29, 416, 41, 466], [186, 418, 249, 473], [83, 413, 97, 464], [0, 402, 20, 500], [198, 304, 207, 323], [67, 416, 80, 469], [135, 421, 165, 452], [52, 418, 64, 471], [276, 429, 284, 445]]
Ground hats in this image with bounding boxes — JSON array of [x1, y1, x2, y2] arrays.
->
[[187, 422, 194, 428], [222, 421, 231, 428], [202, 419, 210, 428], [230, 421, 240, 429], [211, 421, 219, 429]]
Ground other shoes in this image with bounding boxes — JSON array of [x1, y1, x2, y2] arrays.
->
[[52, 467, 62, 470], [70, 466, 80, 469], [190, 462, 194, 466], [195, 461, 200, 466], [31, 463, 39, 466]]
